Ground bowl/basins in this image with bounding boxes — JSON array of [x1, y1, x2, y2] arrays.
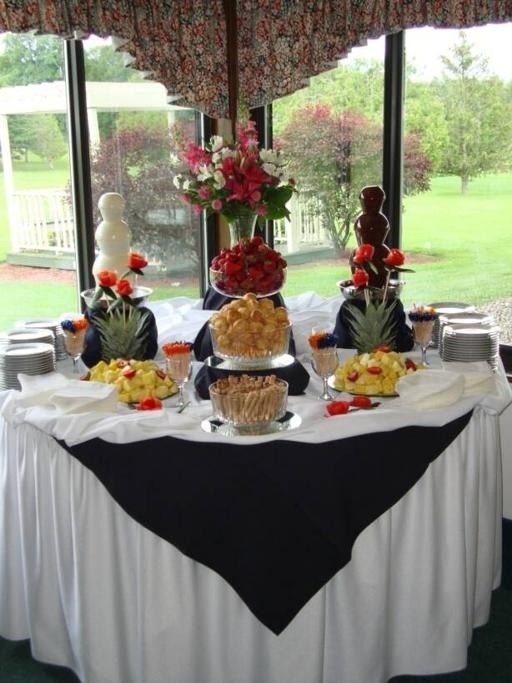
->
[[338, 281, 406, 298], [208, 267, 288, 298], [80, 285, 153, 312], [206, 324, 293, 368], [206, 376, 289, 428]]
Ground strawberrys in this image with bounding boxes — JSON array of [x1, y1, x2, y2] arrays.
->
[[209, 237, 286, 296]]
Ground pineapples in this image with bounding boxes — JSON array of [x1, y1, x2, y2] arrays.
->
[[85, 303, 179, 403], [333, 295, 427, 395]]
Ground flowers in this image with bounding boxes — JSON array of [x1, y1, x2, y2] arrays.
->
[[171, 116, 300, 224]]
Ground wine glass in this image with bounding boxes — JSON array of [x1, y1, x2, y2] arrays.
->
[[310, 352, 339, 402], [411, 321, 436, 368], [61, 331, 87, 375], [165, 357, 191, 414]]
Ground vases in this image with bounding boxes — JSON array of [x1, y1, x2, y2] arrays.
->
[[221, 210, 259, 246]]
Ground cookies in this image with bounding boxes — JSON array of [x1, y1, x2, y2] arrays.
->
[[209, 373, 284, 435]]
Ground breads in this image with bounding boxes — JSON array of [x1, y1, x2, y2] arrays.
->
[[209, 292, 288, 358]]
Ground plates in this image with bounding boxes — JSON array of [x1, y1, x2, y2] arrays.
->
[[325, 375, 409, 399], [0, 319, 64, 393], [428, 300, 500, 363], [201, 410, 303, 441], [203, 353, 295, 373], [113, 387, 180, 405]]
